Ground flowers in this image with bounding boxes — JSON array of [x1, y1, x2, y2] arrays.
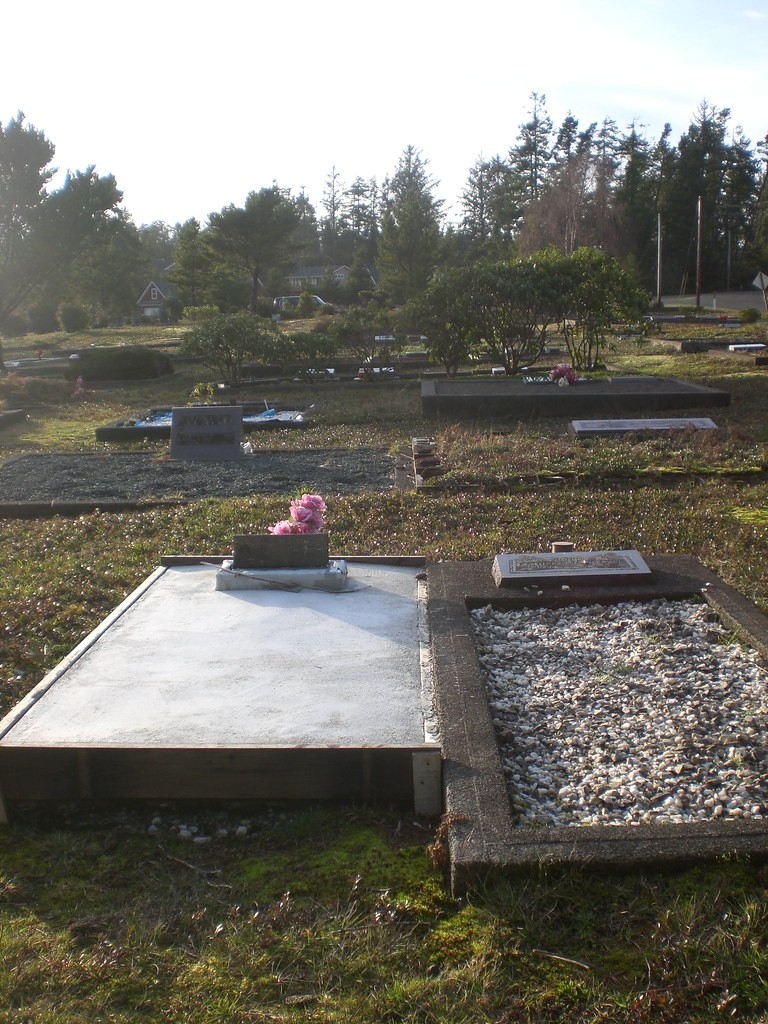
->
[[269, 493, 328, 534], [549, 367, 573, 388]]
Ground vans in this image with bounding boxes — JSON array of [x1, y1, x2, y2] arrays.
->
[[274, 294, 325, 311]]
